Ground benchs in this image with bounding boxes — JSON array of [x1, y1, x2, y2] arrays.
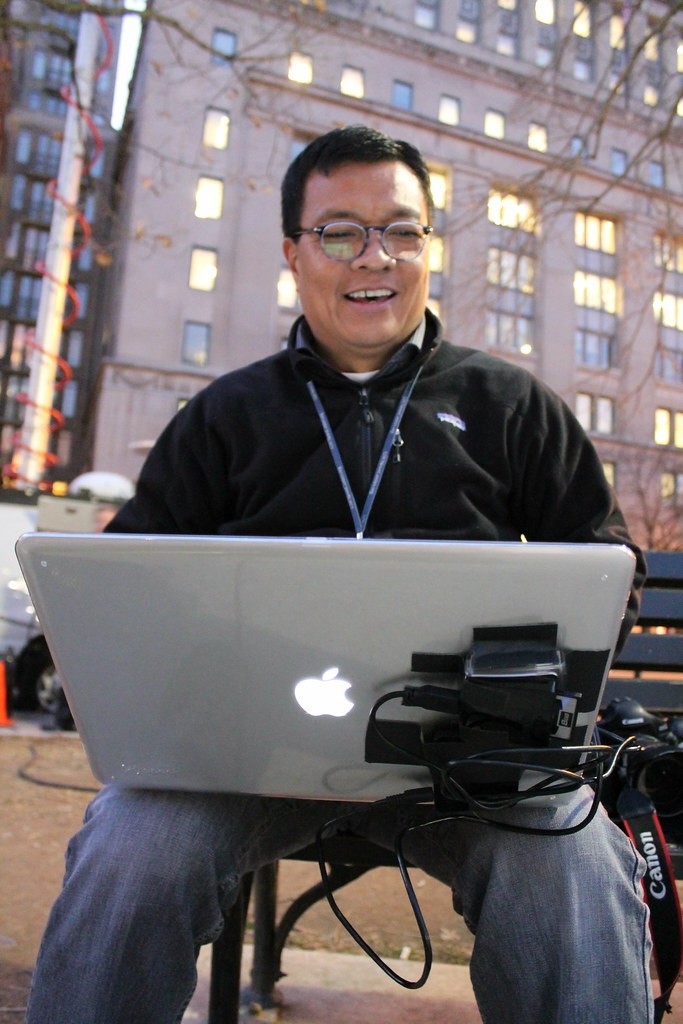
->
[[211, 548, 683, 1024]]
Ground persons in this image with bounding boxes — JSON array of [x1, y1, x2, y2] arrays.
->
[[24, 125, 656, 1024]]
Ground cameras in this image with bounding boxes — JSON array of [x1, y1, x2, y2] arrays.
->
[[593, 695, 683, 823]]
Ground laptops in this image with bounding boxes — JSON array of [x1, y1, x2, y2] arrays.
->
[[15, 530, 637, 807]]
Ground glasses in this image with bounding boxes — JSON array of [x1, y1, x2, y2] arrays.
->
[[286, 221, 433, 262]]
